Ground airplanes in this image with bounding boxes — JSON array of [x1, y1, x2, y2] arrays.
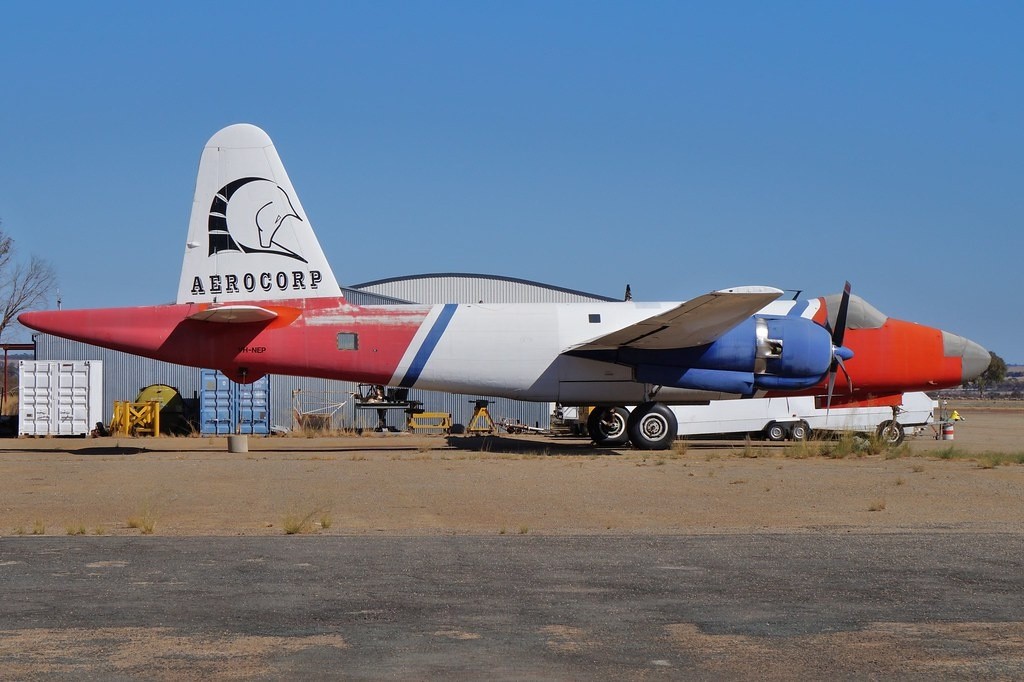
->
[[18, 123, 854, 450], [756, 294, 992, 448]]
